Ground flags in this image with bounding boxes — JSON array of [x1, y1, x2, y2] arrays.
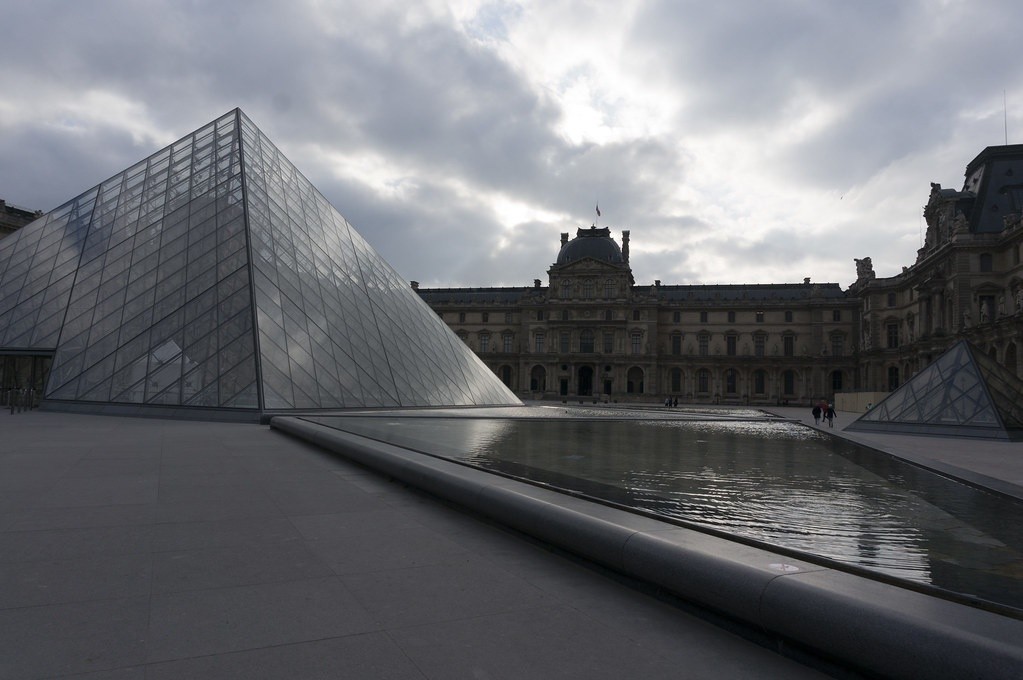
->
[[596, 206, 600, 216]]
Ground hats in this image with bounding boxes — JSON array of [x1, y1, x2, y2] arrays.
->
[[829, 404, 832, 407], [821, 400, 825, 403]]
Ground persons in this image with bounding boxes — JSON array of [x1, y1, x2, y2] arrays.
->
[[772, 343, 778, 355], [998, 296, 1008, 315], [664, 397, 672, 407], [953, 209, 966, 225], [821, 400, 828, 422], [1016, 285, 1023, 310], [688, 342, 694, 353], [865, 403, 871, 410], [963, 305, 970, 326], [714, 342, 721, 354], [812, 403, 821, 424], [674, 395, 678, 406], [743, 342, 750, 354], [827, 404, 837, 428], [981, 300, 989, 321]]
